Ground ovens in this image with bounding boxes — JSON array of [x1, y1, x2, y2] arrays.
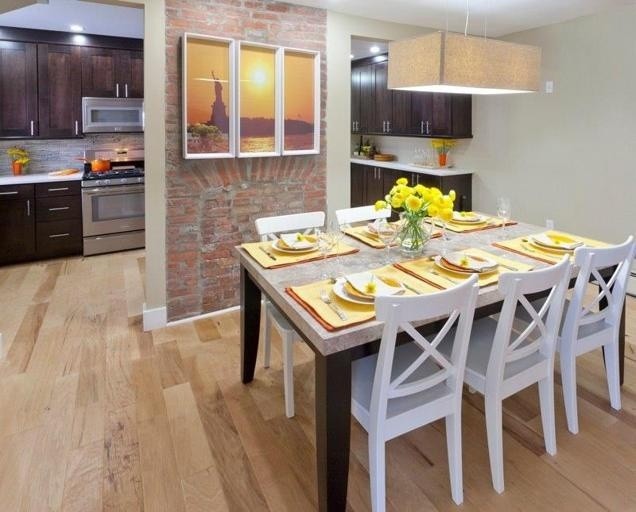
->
[[81, 184, 145, 256]]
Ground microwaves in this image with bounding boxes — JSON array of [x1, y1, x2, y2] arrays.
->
[[82, 96, 145, 133]]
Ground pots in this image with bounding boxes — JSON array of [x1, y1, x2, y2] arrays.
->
[[73, 156, 112, 172]]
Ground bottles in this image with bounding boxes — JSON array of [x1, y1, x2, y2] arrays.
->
[[352, 136, 378, 157]]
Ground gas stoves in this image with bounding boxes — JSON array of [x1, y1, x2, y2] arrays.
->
[[80, 167, 145, 188]]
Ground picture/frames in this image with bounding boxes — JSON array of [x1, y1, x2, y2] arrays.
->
[[236, 39, 281, 159], [181, 32, 236, 162], [282, 46, 322, 157]]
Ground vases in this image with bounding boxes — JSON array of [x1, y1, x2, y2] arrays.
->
[[439, 152, 447, 167]]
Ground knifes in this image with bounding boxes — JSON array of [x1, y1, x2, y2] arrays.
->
[[259, 246, 276, 261]]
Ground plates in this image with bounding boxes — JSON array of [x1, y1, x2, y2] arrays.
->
[[270, 237, 320, 253], [434, 254, 497, 274], [526, 233, 573, 253], [449, 213, 487, 224], [364, 226, 383, 237], [332, 275, 405, 305]]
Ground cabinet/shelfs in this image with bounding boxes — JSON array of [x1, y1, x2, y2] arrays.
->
[[351, 64, 376, 136], [350, 158, 473, 213], [37, 43, 83, 140], [412, 93, 474, 140], [0, 184, 36, 268], [34, 179, 83, 261], [376, 60, 412, 138], [81, 47, 144, 99], [0, 38, 39, 141]]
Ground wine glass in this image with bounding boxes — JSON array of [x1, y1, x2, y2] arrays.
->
[[410, 144, 430, 166], [377, 224, 397, 264], [422, 217, 458, 252], [317, 218, 346, 275], [495, 197, 511, 239]]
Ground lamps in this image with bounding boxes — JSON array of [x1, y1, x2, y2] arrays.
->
[[386, 2, 541, 95]]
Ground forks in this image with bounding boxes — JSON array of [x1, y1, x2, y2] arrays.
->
[[318, 286, 347, 322]]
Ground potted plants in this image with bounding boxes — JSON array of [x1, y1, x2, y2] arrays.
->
[[4, 145, 30, 177]]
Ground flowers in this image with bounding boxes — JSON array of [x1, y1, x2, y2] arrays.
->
[[429, 138, 460, 155], [374, 177, 458, 252]]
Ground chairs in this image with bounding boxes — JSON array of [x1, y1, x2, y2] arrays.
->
[[424, 251, 575, 495], [254, 210, 329, 420], [334, 199, 393, 226], [349, 269, 485, 512], [491, 233, 634, 435]]
